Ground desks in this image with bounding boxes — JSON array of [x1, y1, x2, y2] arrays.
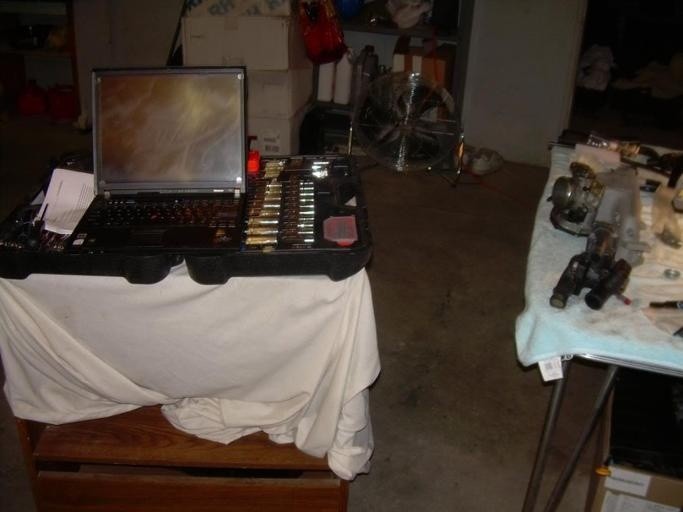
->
[[523, 142, 682, 507]]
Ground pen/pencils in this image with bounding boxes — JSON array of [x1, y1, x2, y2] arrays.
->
[[614, 292, 631, 305]]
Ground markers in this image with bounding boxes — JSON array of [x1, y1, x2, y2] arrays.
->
[[649, 300, 683, 310]]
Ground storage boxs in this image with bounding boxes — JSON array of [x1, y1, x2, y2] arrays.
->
[[187, 1, 293, 17], [246, 106, 312, 158], [587, 381, 681, 507], [246, 59, 314, 117], [181, 16, 306, 71]]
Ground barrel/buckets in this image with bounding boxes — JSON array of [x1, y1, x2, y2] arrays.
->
[[352, 44, 378, 107], [333, 48, 352, 106]]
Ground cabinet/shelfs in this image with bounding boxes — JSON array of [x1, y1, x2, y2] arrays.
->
[[0, 275, 383, 512]]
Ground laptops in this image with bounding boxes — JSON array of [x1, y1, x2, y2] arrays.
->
[[64, 66, 247, 253]]
